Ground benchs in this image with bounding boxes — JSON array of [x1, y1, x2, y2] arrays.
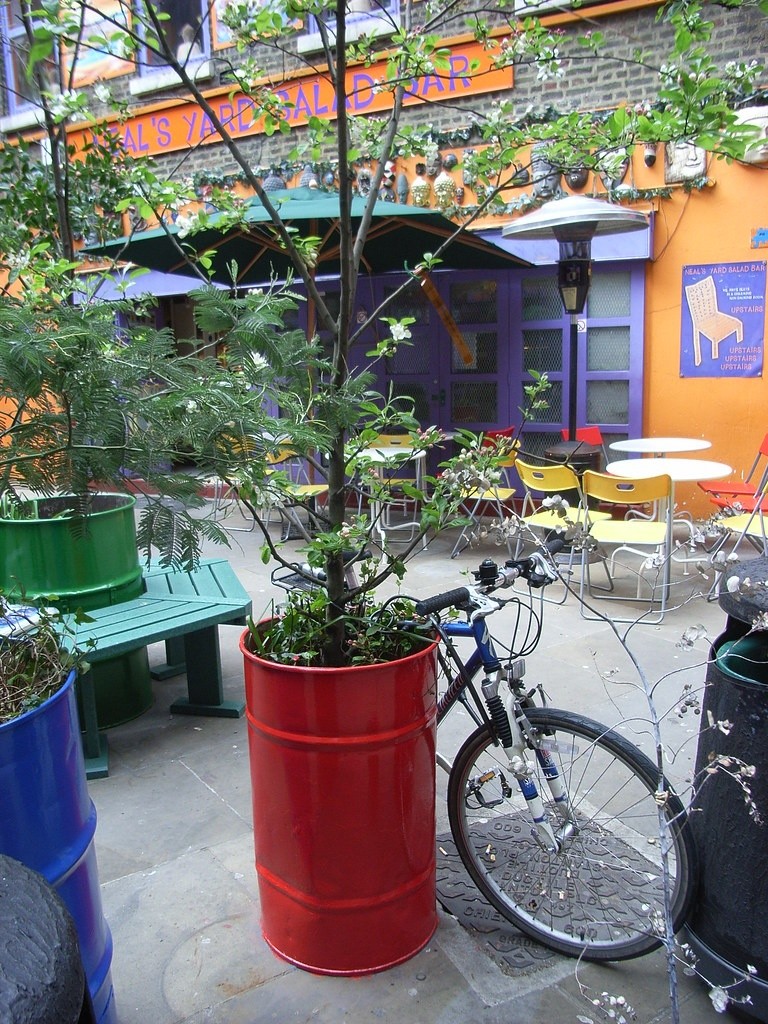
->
[[29, 555, 253, 779]]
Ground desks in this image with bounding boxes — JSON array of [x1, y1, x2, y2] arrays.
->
[[609, 437, 712, 553], [325, 447, 429, 564], [606, 458, 733, 597]]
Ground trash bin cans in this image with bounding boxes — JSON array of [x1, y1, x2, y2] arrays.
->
[[678, 549, 768, 1024]]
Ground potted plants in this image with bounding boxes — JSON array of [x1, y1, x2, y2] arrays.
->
[[0, 0, 768, 976], [0, 285, 378, 733], [0, 574, 117, 1024]]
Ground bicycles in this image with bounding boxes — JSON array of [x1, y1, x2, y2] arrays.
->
[[273, 534, 702, 964]]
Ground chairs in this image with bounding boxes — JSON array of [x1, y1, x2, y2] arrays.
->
[[710, 465, 768, 564], [212, 433, 283, 532], [358, 433, 428, 542], [561, 427, 636, 518], [435, 425, 517, 519], [510, 458, 614, 605], [697, 432, 768, 553], [450, 440, 525, 561], [706, 481, 768, 603], [578, 470, 673, 625], [263, 439, 329, 546]]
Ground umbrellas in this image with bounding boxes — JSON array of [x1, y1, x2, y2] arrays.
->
[[78, 186, 538, 487]]
